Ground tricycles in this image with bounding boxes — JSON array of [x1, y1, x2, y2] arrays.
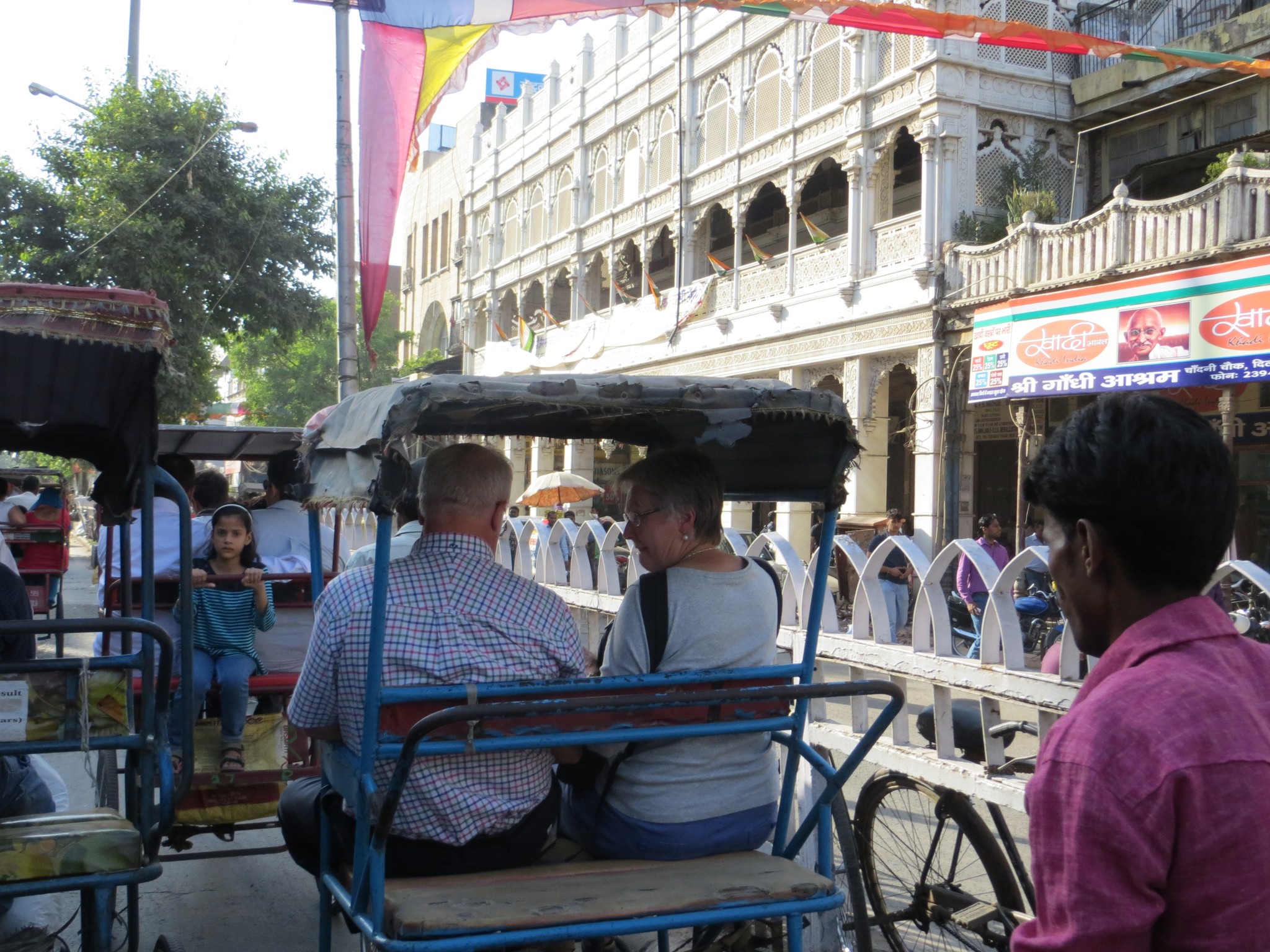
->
[[0, 273, 908, 952]]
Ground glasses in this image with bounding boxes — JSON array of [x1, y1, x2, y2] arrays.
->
[[263, 479, 273, 490], [622, 504, 673, 528]]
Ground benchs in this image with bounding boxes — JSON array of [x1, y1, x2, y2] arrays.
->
[[353, 847, 841, 944], [125, 665, 304, 700], [0, 802, 141, 886]]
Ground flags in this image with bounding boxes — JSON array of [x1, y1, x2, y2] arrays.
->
[[579, 294, 598, 316], [800, 213, 832, 245], [494, 322, 509, 342], [612, 278, 638, 305], [746, 235, 774, 265], [644, 272, 661, 309], [461, 340, 477, 355], [706, 252, 732, 277], [519, 316, 535, 354], [541, 307, 563, 329]]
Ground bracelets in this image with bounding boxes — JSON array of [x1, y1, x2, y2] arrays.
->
[[886, 567, 891, 577]]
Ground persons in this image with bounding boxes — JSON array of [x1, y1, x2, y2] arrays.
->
[[497, 499, 627, 588], [551, 445, 783, 952], [866, 508, 915, 644], [1017, 387, 1269, 952], [758, 510, 776, 560], [1024, 519, 1050, 631], [344, 457, 430, 574], [810, 510, 825, 559], [275, 443, 590, 936], [1123, 308, 1189, 362], [0, 471, 78, 952], [153, 500, 277, 776], [91, 455, 351, 724], [956, 513, 1014, 660]]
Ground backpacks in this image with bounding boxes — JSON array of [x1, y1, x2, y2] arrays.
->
[[596, 557, 783, 671]]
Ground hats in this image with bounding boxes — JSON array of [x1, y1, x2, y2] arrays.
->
[[39, 487, 63, 509]]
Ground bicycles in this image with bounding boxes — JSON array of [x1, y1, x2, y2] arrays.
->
[[855, 697, 1046, 952]]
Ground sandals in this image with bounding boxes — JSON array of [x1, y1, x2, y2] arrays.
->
[[219, 743, 245, 773], [155, 751, 184, 775]]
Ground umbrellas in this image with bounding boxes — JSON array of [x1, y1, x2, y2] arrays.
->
[[515, 471, 606, 507]]
